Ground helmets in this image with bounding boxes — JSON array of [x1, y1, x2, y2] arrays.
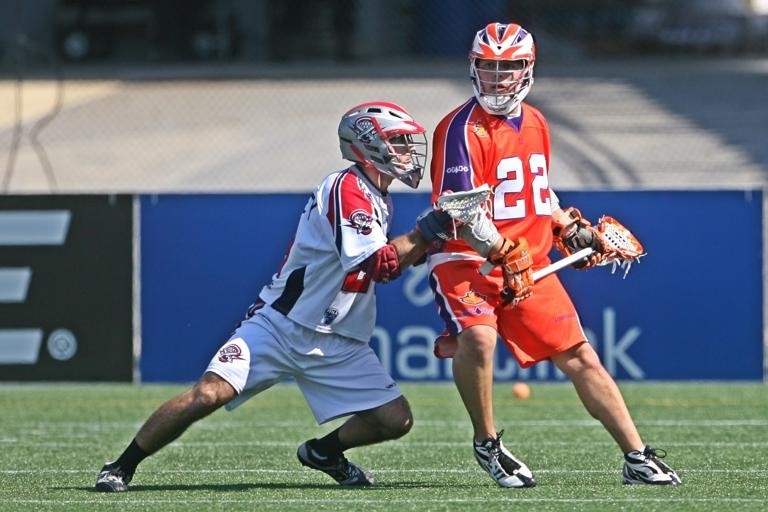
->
[[337, 101, 427, 188], [467, 21, 538, 110]]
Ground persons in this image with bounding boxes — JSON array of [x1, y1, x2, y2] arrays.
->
[[94, 100, 497, 494], [429, 21, 680, 487]]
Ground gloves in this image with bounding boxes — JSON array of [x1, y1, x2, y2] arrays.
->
[[477, 236, 536, 311], [552, 205, 605, 272], [415, 188, 467, 244]]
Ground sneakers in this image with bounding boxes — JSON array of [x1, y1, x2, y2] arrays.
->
[[621, 444, 682, 485], [296, 437, 371, 485], [472, 432, 536, 488], [95, 457, 137, 492]]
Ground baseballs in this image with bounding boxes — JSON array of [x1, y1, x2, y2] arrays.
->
[[512, 383, 531, 399]]
